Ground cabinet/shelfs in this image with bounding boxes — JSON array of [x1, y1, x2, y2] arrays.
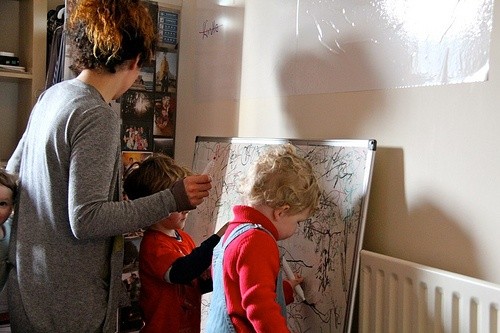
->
[[0, 0, 48, 171]]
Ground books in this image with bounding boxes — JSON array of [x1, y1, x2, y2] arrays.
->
[[0, 65, 26, 73]]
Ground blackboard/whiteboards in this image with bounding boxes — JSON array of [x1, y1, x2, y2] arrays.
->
[[182, 135, 378, 333]]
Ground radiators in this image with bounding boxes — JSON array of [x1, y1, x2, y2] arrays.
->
[[358, 250, 500, 333]]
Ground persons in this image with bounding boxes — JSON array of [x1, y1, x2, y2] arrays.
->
[[5, 1, 213, 333], [124, 126, 149, 151], [156, 109, 170, 133], [0, 166, 22, 296], [160, 54, 169, 93], [123, 154, 231, 333], [202, 149, 322, 333]]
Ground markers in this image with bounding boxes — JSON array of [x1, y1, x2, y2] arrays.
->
[[283, 256, 307, 304], [201, 157, 216, 176]]
